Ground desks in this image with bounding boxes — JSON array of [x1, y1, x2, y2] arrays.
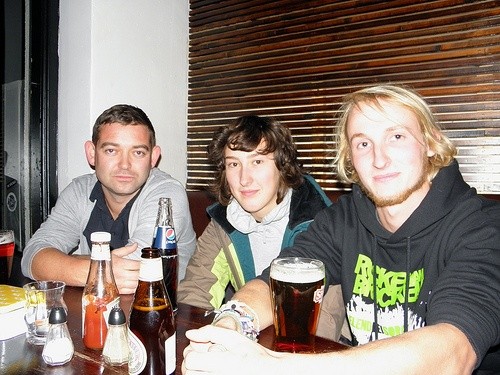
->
[[0, 285, 351, 375]]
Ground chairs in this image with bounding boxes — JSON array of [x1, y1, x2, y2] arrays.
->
[[0, 241, 14, 277]]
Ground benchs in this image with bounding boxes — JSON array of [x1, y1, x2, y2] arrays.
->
[[188, 190, 500, 241]]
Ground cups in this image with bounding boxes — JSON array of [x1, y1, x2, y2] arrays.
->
[[24, 280, 68, 345], [269, 257, 325, 354], [0, 230, 15, 279]]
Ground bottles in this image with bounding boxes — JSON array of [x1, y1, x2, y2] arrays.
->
[[128, 247, 176, 375], [153, 198, 177, 305], [82, 232, 120, 349], [42, 307, 74, 366], [103, 308, 129, 366]]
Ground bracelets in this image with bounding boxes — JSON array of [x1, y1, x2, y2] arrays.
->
[[213, 301, 259, 345]]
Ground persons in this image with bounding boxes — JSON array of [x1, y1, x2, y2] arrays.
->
[[21, 105, 197, 296], [175, 115, 332, 313], [180, 86, 500, 375]]
